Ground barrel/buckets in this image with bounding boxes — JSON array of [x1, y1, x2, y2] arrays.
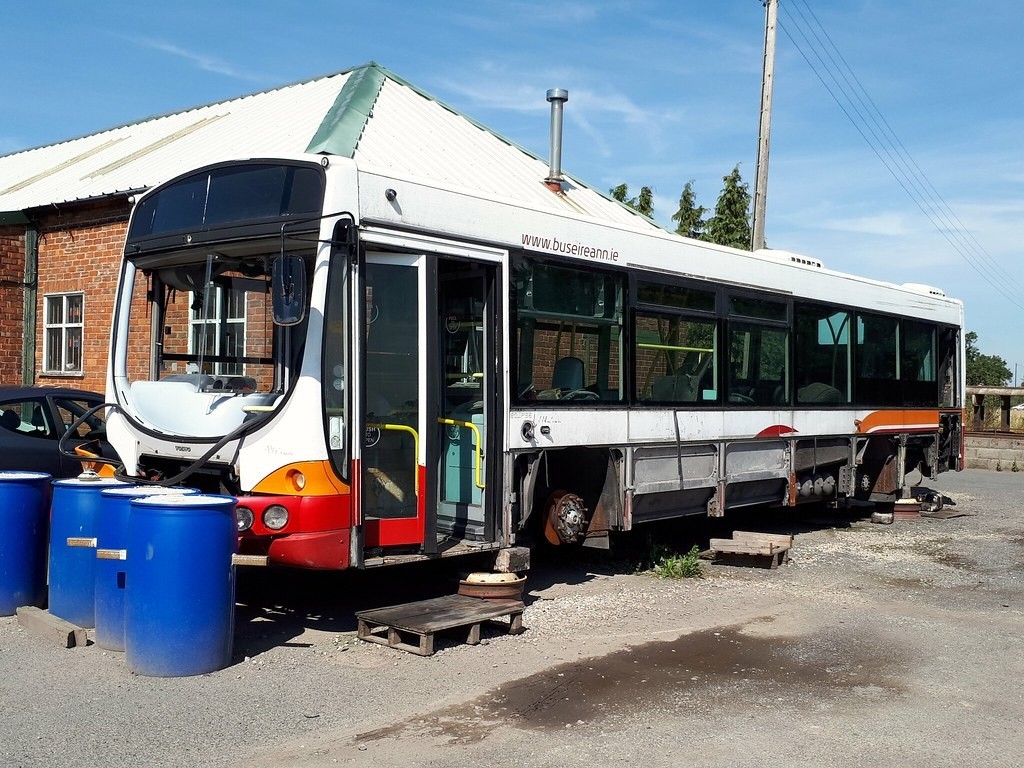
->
[[93, 486, 203, 652], [123, 494, 239, 677], [48, 476, 136, 629], [0, 469, 53, 618]]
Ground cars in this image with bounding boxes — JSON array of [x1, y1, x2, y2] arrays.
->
[[0, 384, 124, 477]]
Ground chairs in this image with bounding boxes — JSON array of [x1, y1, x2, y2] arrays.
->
[[652, 375, 694, 401], [2, 410, 21, 430], [30, 406, 47, 438], [551, 358, 598, 400]]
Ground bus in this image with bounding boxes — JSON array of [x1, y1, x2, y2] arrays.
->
[[58, 153, 969, 576]]
